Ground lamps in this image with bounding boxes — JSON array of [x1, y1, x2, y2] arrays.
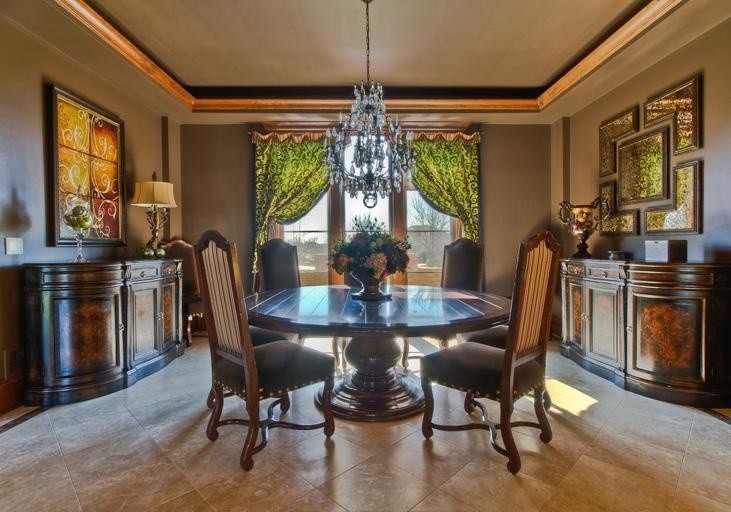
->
[[128, 171, 178, 258], [319, 0, 420, 208]]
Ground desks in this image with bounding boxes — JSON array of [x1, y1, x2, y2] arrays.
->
[[245, 284, 511, 423]]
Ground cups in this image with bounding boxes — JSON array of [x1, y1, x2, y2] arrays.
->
[[608, 250, 623, 260]]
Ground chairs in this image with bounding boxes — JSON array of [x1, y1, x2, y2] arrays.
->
[[332, 269, 409, 370], [207, 324, 288, 409], [256, 239, 301, 292], [155, 234, 205, 347], [464, 325, 552, 410], [441, 238, 485, 293], [194, 230, 335, 471], [419, 229, 561, 474]]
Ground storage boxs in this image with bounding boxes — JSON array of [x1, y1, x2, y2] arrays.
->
[[645, 240, 687, 263]]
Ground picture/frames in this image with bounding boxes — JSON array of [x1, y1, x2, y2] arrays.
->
[[598, 72, 703, 238], [44, 82, 127, 247]]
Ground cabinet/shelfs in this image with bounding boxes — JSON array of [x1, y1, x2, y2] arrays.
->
[[557, 257, 730, 410], [22, 257, 181, 407]]
